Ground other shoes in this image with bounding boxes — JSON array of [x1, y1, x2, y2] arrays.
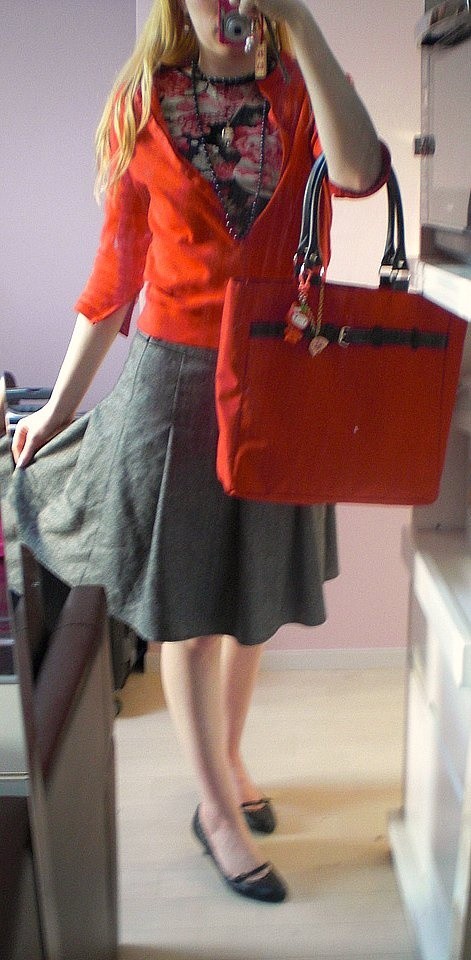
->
[[240, 795, 275, 832], [192, 803, 285, 903]]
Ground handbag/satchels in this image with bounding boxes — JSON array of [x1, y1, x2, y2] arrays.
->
[[213, 154, 466, 506]]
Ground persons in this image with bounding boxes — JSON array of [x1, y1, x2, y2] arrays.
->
[[12, 0, 389, 905]]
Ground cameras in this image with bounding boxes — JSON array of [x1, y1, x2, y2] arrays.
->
[[217, 0, 256, 47]]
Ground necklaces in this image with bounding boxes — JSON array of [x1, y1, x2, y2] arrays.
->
[[201, 64, 258, 145], [191, 53, 274, 240]]
